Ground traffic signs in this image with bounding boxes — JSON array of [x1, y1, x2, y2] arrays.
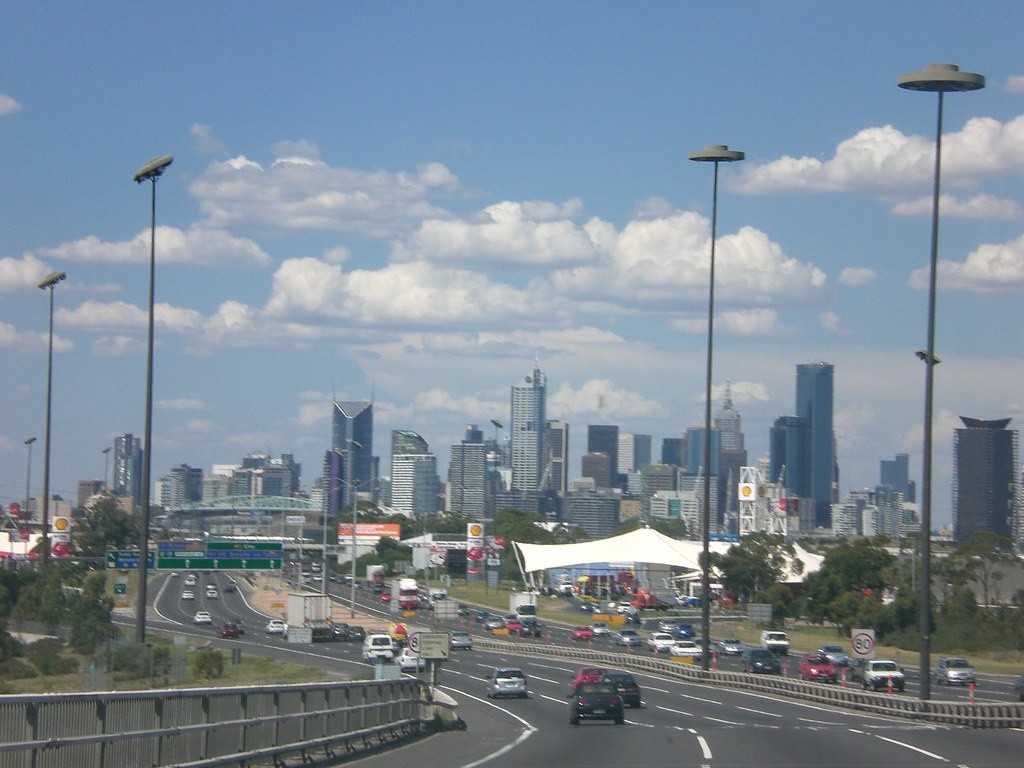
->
[[105, 550, 155, 570], [155, 541, 282, 571]]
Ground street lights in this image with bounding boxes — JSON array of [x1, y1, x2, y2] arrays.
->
[[490, 419, 504, 519], [321, 476, 386, 618], [312, 488, 327, 594], [132, 154, 174, 643], [689, 144, 746, 679], [37, 271, 66, 564], [896, 62, 987, 720], [24, 437, 113, 530]]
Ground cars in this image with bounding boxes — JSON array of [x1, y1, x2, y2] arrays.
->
[[559, 581, 790, 724], [170, 570, 246, 639], [265, 592, 528, 701], [301, 564, 447, 610], [682, 597, 702, 608], [798, 645, 1024, 702]]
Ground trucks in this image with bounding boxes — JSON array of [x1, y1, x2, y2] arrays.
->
[[510, 592, 537, 620]]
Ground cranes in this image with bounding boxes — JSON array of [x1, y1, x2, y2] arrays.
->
[[688, 464, 787, 536]]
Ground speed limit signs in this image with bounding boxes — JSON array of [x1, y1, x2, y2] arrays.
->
[[408, 632, 422, 653], [852, 633, 875, 655]]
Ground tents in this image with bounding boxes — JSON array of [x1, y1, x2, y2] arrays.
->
[[509, 521, 828, 606]]
